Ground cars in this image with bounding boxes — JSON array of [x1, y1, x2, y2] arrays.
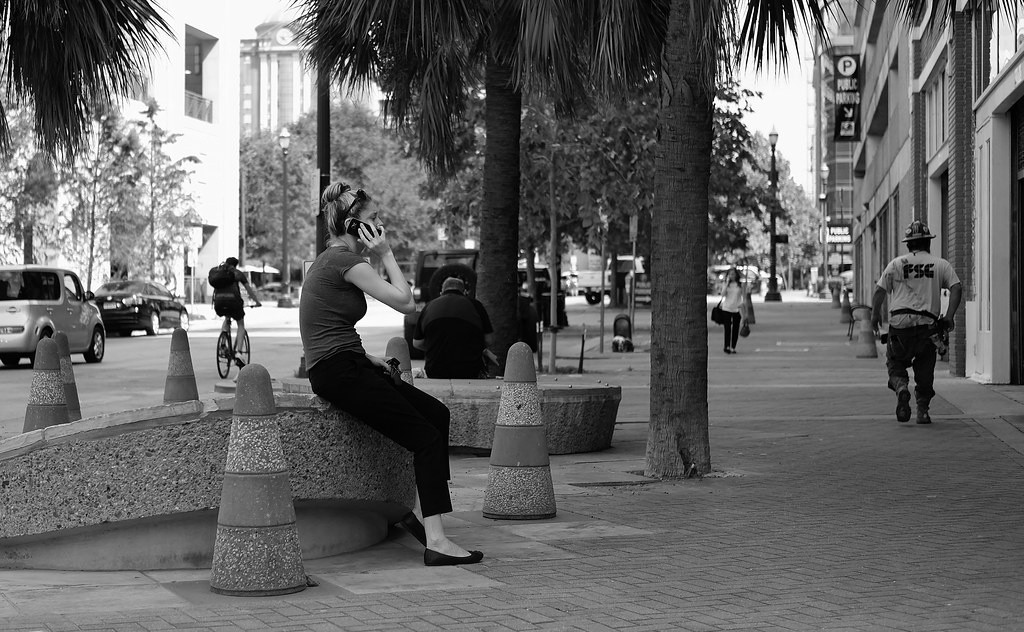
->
[[0, 265, 106, 367], [89, 280, 189, 336], [518, 266, 566, 326], [707, 266, 783, 295], [404, 249, 536, 360], [840, 270, 853, 292]]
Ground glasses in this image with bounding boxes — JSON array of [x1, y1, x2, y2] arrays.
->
[[345, 188, 367, 217]]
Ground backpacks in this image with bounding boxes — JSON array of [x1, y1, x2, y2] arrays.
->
[[209, 263, 235, 288]]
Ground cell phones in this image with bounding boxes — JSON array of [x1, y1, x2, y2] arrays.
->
[[347, 219, 382, 241]]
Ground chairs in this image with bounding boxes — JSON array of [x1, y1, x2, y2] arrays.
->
[[847, 305, 880, 340]]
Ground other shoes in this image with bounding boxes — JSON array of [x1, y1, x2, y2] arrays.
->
[[219, 346, 228, 356], [235, 352, 247, 367], [724, 348, 731, 353], [401, 509, 427, 547], [731, 348, 736, 353], [424, 548, 483, 566]]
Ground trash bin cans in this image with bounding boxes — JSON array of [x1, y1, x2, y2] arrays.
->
[[612, 314, 634, 352]]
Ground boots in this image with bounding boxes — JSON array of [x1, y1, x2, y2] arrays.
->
[[888, 370, 912, 422], [914, 386, 935, 424]]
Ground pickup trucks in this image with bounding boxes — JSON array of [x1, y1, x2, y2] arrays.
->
[[570, 255, 645, 304]]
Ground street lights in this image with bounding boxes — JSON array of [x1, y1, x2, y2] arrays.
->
[[765, 126, 783, 302], [819, 162, 832, 299], [278, 127, 293, 308]]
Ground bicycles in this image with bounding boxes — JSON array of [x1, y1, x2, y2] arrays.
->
[[216, 304, 262, 379]]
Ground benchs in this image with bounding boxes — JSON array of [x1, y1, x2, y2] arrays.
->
[[0, 392, 418, 573], [216, 378, 622, 455]]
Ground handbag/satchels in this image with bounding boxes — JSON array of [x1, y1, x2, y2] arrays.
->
[[482, 348, 502, 378], [711, 307, 724, 324], [740, 319, 750, 337]]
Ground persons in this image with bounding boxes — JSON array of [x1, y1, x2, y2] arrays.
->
[[299, 183, 484, 567], [411, 278, 495, 380], [720, 268, 749, 354], [869, 221, 964, 423], [212, 256, 261, 367]]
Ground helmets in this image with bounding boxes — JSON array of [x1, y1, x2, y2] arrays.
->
[[902, 222, 936, 243]]
